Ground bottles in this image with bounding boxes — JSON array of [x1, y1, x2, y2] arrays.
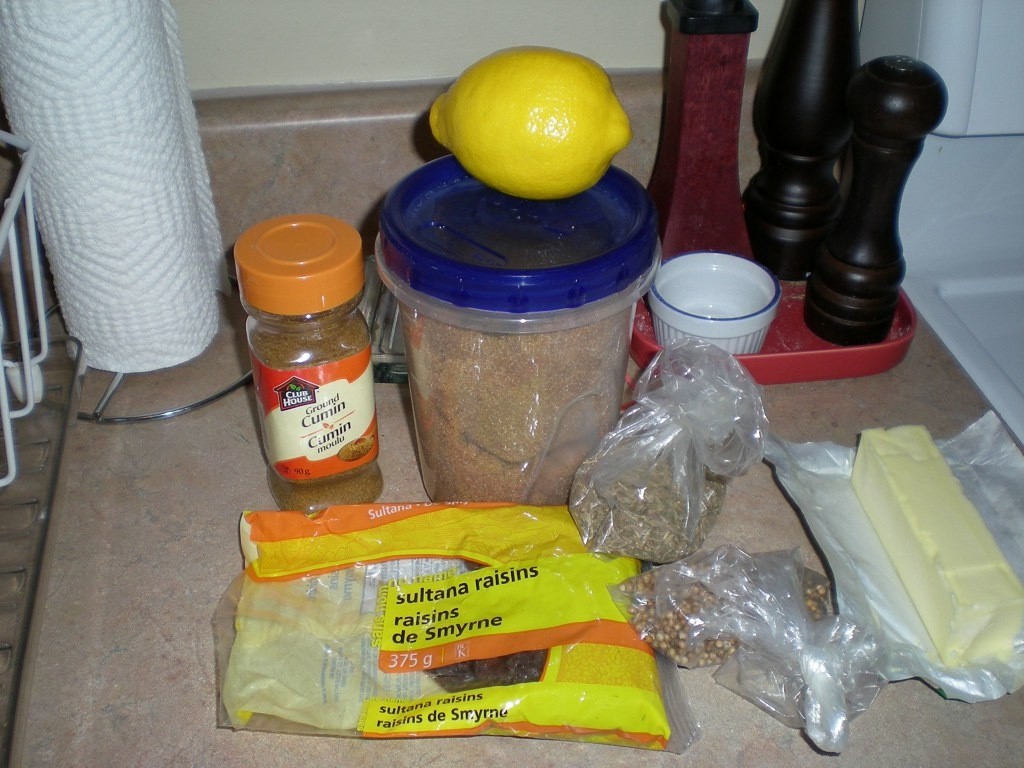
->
[[804, 54, 949, 345], [233, 213, 383, 511], [741, 0, 861, 279], [376, 158, 663, 506]]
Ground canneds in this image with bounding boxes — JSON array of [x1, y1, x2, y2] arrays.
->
[[233, 214, 384, 515]]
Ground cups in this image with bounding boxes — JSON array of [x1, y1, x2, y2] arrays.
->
[[647, 250, 781, 356]]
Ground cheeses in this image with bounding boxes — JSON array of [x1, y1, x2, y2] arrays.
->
[[851, 423, 1024, 667]]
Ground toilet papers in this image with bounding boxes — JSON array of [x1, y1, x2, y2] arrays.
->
[[0, 0, 235, 375]]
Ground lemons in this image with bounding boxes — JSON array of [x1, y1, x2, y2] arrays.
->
[[430, 46, 632, 199]]
